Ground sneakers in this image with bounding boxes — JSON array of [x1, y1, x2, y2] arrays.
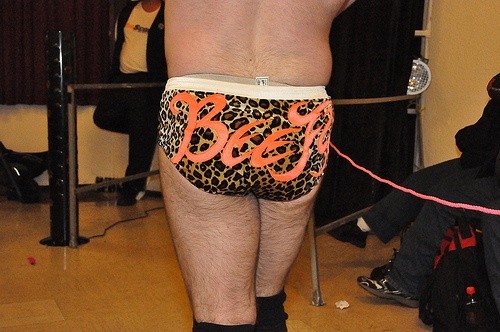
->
[[327, 219, 369, 248], [371, 263, 391, 280], [357, 274, 420, 308]]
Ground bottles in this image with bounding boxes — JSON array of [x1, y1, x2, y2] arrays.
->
[[463, 286, 486, 332]]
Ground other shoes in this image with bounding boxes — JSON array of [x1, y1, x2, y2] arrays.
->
[[117, 182, 145, 206]]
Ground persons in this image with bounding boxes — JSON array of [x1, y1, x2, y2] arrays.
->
[[158, 0, 355, 332], [93, 0, 168, 202], [330, 73, 500, 306]]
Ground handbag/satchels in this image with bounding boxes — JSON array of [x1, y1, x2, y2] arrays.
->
[[420, 222, 500, 332], [0, 141, 49, 204]]
